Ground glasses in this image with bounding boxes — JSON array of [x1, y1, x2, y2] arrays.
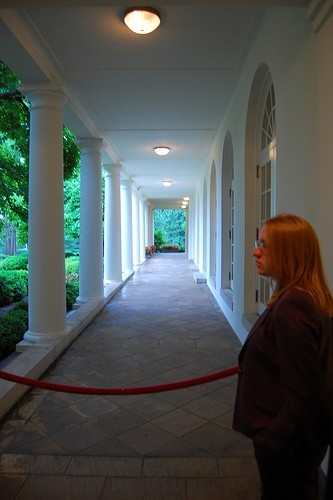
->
[[254, 241, 270, 250]]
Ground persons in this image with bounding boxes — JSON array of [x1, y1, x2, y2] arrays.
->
[[232, 214, 333, 500]]
